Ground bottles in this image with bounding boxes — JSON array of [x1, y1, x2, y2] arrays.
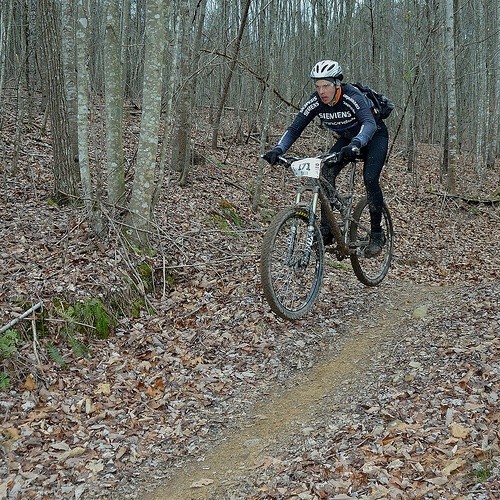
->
[[364, 95, 374, 108]]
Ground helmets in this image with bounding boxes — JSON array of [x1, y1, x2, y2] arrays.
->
[[310, 59, 342, 79]]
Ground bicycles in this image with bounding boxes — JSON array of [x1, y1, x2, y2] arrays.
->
[[260, 148, 394, 321]]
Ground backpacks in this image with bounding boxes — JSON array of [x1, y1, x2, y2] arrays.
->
[[318, 82, 395, 138]]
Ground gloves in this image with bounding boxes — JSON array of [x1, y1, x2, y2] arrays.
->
[[263, 148, 283, 166], [338, 140, 361, 163]]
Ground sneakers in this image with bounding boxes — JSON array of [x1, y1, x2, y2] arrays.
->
[[313, 221, 344, 246], [364, 226, 387, 258]]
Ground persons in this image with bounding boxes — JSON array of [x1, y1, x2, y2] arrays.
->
[[265, 60, 389, 257]]
